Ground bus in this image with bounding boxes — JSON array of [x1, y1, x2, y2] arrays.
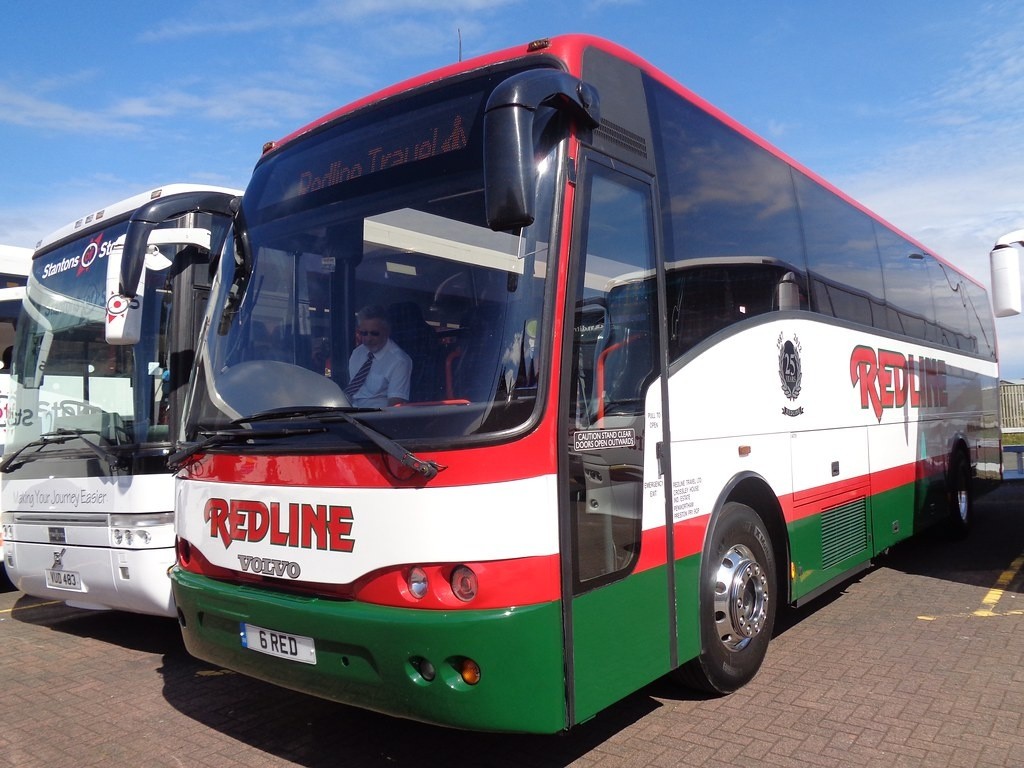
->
[[0, 183, 660, 619], [118, 32, 1004, 735]]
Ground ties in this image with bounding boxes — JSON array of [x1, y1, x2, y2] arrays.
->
[[343, 352, 374, 398]]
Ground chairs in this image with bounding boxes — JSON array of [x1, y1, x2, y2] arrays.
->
[[252, 320, 279, 362], [386, 302, 447, 405], [2, 345, 14, 370], [271, 323, 303, 363]]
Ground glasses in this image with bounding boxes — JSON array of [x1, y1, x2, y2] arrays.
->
[[356, 328, 385, 338]]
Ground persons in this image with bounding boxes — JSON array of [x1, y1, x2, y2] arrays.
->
[[342, 306, 412, 408]]
